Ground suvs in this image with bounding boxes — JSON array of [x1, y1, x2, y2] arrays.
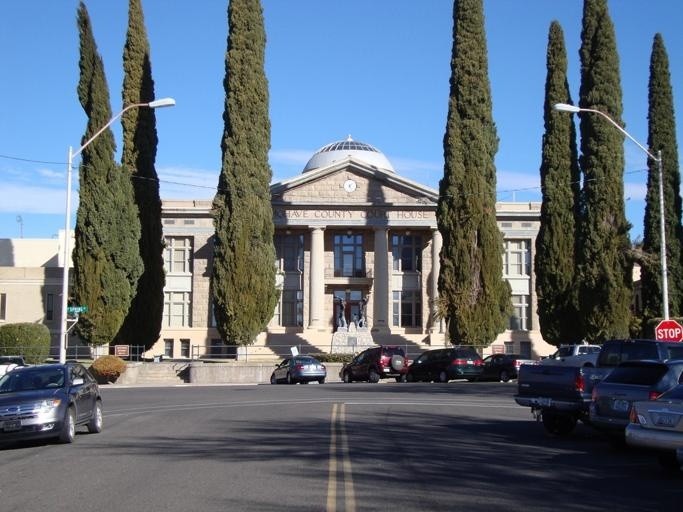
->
[[343, 345, 408, 384]]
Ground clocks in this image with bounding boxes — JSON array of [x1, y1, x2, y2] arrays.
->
[[343, 178, 356, 193]]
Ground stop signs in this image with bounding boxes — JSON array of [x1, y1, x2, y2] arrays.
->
[[654, 318, 683, 342]]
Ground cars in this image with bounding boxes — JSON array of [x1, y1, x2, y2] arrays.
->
[[412, 346, 484, 383], [0, 360, 107, 443], [514, 339, 682, 452], [271, 353, 329, 385], [481, 350, 539, 382]]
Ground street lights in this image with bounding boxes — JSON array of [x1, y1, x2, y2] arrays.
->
[[58, 97, 179, 364], [554, 99, 669, 320]]
